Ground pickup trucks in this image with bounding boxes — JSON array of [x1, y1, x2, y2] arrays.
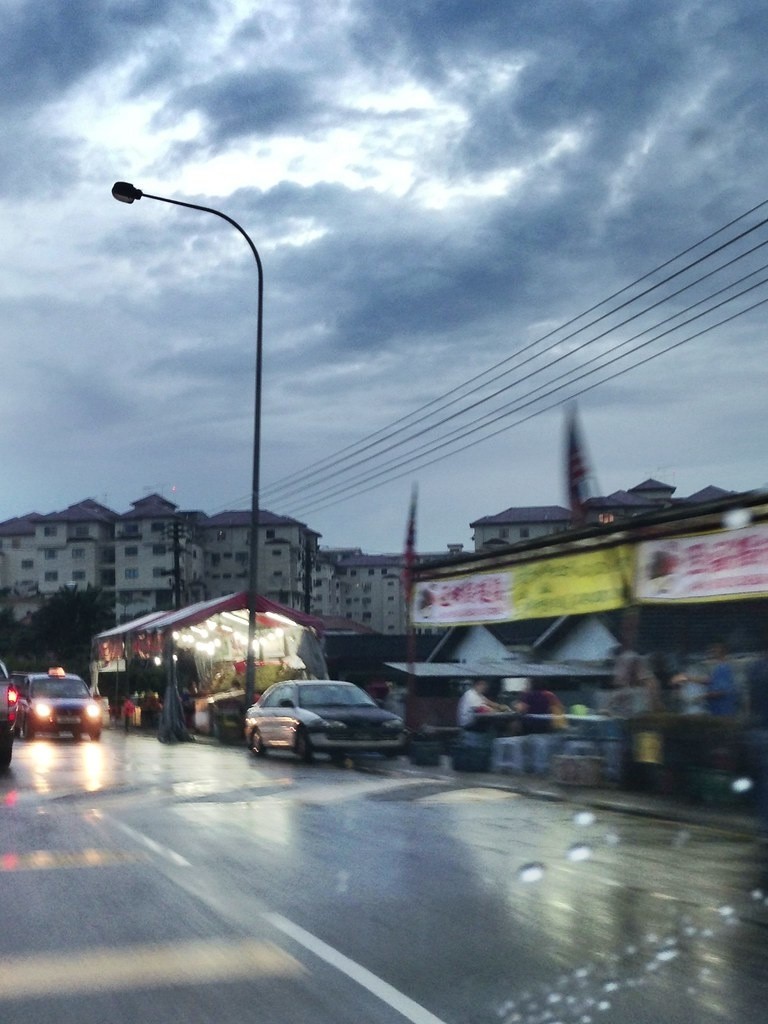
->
[[0, 660, 18, 767]]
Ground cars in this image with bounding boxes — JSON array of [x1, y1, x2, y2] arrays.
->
[[241, 678, 409, 771], [17, 667, 105, 743]]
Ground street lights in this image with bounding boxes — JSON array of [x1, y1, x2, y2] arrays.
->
[[110, 181, 261, 721]]
